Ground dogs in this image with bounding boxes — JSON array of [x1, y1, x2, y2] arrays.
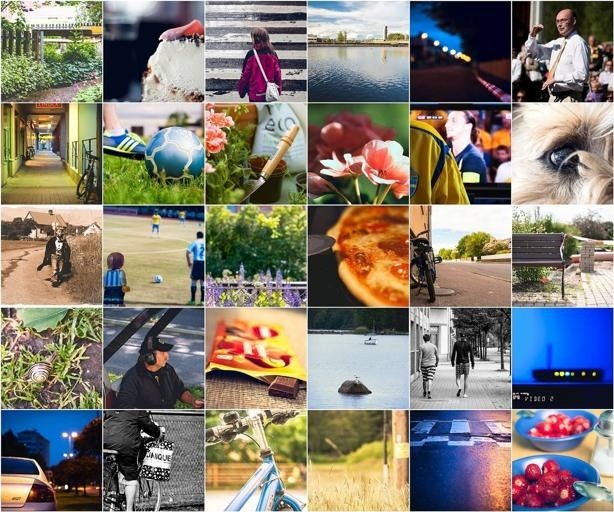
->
[[511, 102, 612, 205]]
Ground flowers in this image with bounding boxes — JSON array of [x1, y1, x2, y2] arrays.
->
[[308, 112, 410, 204]]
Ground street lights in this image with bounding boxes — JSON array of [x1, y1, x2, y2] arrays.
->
[[62, 453, 74, 460], [61, 431, 77, 459]]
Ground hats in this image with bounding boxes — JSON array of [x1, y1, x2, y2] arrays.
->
[[141, 338, 173, 351], [460, 333, 467, 339]]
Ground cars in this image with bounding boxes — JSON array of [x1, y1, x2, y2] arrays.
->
[[0, 455, 57, 511]]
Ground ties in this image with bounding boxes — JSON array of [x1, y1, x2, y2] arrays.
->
[[548, 40, 566, 77]]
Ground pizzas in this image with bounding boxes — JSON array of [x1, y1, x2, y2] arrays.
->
[[328, 206, 409, 307]]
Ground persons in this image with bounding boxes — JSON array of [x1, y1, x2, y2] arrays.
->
[[185, 231, 206, 305], [37, 226, 72, 286], [178, 210, 185, 223], [150, 208, 161, 238], [103, 252, 127, 306], [418, 335, 439, 399], [103, 410, 163, 511], [116, 337, 204, 409], [452, 335, 475, 398], [238, 27, 283, 102], [512, 8, 613, 102], [410, 108, 512, 204], [103, 104, 146, 155]]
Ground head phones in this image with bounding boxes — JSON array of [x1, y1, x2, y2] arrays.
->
[[145, 336, 156, 364]]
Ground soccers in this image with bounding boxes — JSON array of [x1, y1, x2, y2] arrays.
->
[[146, 127, 204, 187], [152, 275, 162, 282]]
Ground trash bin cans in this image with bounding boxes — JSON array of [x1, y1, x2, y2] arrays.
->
[[580, 242, 595, 272]]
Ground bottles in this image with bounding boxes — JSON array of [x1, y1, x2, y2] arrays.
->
[[586, 409, 613, 477]]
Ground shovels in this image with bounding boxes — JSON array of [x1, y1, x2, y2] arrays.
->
[[238, 124, 299, 204]]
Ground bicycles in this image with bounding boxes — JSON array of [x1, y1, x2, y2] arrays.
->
[[409, 229, 442, 302], [26, 146, 35, 160], [75, 148, 99, 204], [100, 429, 173, 511], [203, 410, 307, 511]]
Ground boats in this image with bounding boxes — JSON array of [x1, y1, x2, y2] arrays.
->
[[364, 336, 378, 347]]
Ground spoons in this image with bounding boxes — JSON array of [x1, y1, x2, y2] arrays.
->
[[571, 480, 614, 505], [516, 409, 536, 418]]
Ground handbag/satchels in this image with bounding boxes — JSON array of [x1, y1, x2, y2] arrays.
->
[[138, 439, 175, 481], [265, 82, 280, 102]]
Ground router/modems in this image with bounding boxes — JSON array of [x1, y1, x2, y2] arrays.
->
[[532, 343, 605, 382]]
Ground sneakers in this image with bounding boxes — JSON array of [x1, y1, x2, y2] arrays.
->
[[428, 390, 431, 398], [103, 129, 145, 161], [457, 388, 461, 396]]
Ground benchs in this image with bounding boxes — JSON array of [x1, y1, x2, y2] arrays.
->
[[511, 232, 567, 298]]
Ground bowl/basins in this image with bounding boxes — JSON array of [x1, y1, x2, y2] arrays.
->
[[515, 409, 598, 451], [512, 453, 602, 510]]
